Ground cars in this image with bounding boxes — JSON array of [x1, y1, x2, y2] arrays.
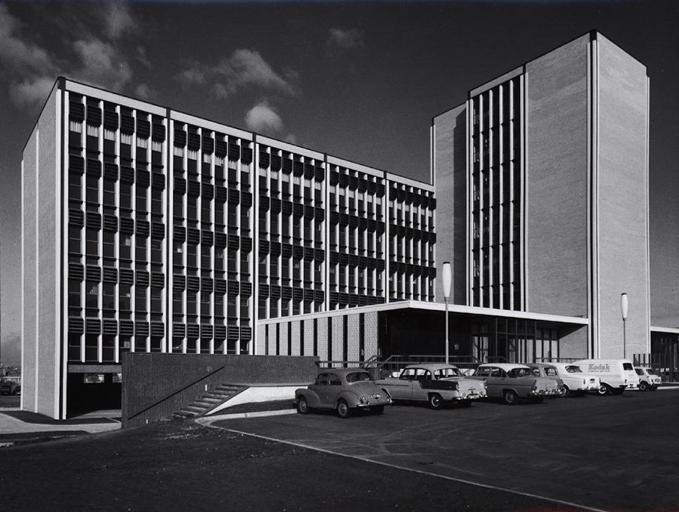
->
[[293, 367, 392, 416], [374, 362, 486, 409], [0, 381, 20, 396], [465, 357, 663, 406]]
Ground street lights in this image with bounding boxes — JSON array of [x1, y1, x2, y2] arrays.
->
[[620, 292, 629, 358], [440, 260, 453, 363]]
